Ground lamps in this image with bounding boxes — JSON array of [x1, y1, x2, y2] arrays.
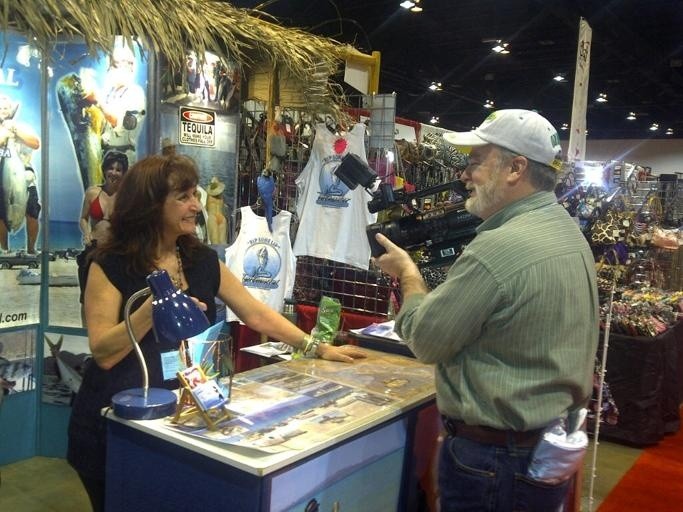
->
[[113, 272, 209, 420]]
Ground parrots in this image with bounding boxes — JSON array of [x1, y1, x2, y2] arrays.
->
[[256, 166, 278, 237]]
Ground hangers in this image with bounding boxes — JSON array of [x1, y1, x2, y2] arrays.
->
[[306, 104, 374, 136], [231, 173, 298, 222]]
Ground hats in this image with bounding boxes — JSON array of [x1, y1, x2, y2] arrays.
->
[[443, 109, 563, 171]]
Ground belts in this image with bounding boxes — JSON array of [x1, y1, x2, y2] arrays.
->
[[442, 416, 538, 448]]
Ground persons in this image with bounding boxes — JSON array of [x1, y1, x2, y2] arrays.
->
[[370, 109, 601, 512], [66, 153, 368, 512], [79, 152, 128, 244], [87, 48, 147, 152], [0, 96, 41, 255]]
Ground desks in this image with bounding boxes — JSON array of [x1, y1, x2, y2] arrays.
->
[[102, 343, 436, 511], [598, 315, 683, 451]]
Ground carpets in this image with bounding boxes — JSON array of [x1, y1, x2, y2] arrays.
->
[[598, 429, 683, 511]]
